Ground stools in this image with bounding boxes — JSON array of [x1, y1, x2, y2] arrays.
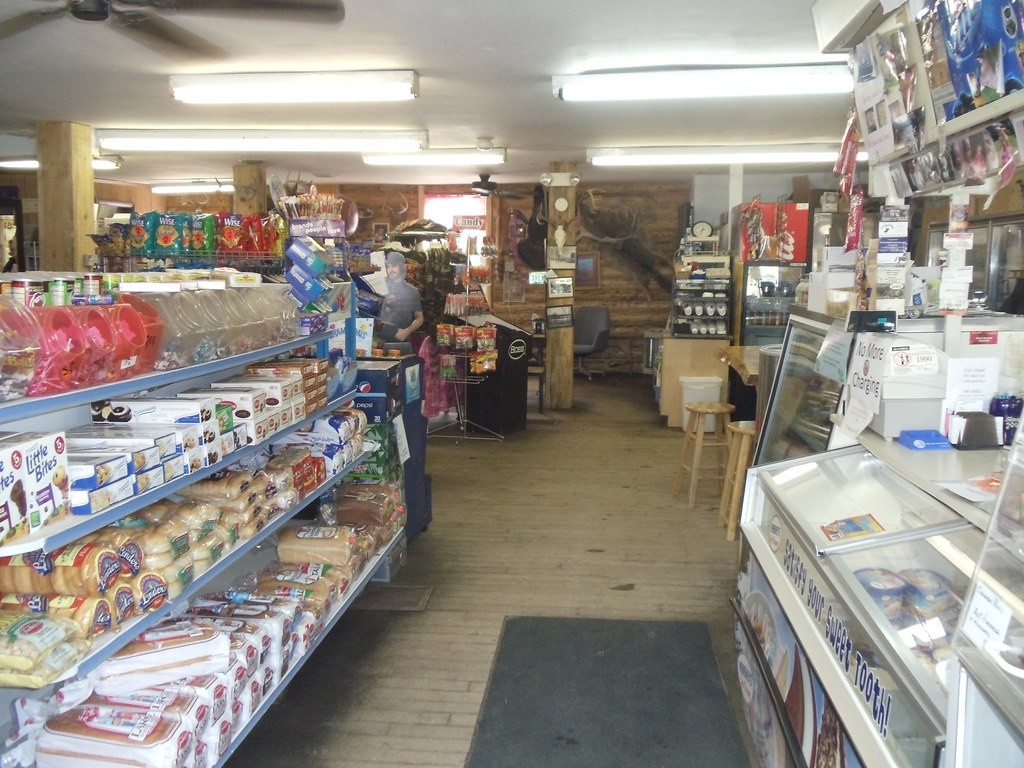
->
[[669, 401, 736, 510], [718, 420, 757, 541], [527, 366, 545, 414]]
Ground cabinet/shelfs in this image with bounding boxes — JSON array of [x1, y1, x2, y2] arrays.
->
[[671, 275, 731, 338], [0, 329, 408, 768], [659, 334, 734, 429]]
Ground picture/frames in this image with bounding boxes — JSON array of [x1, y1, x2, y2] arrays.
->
[[546, 276, 575, 299], [545, 304, 574, 329], [574, 250, 603, 291], [546, 244, 578, 270]]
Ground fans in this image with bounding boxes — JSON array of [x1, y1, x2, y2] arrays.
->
[[471, 174, 497, 194]]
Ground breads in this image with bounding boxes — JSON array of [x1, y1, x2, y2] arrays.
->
[[0, 409, 405, 768]]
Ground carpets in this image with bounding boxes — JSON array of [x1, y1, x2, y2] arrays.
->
[[462, 615, 752, 768]]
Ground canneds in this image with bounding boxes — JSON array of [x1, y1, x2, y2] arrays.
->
[[0, 273, 211, 308], [746, 310, 788, 326]]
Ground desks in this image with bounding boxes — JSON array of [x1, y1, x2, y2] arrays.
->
[[523, 328, 546, 367], [713, 344, 765, 420]]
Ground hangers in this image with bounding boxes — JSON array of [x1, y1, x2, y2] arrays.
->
[[376, 233, 453, 261]]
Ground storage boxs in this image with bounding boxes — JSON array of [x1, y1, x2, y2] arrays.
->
[[98, 199, 133, 219], [284, 219, 347, 311], [332, 360, 404, 485], [683, 255, 729, 271], [295, 313, 329, 336], [866, 311, 1024, 441], [0, 355, 328, 547], [731, 175, 882, 316]]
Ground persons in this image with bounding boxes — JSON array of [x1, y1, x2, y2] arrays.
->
[[375, 226, 387, 243], [380, 252, 424, 342]]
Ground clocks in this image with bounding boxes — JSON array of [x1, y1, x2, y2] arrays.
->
[[692, 220, 713, 237]]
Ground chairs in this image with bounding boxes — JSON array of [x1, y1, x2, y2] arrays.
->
[[571, 300, 610, 382]]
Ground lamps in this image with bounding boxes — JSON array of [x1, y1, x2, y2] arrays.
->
[[0, 155, 125, 171], [551, 62, 853, 103], [167, 70, 420, 106], [149, 182, 236, 194], [539, 172, 581, 186], [93, 127, 430, 154], [584, 142, 870, 167], [361, 146, 506, 167]]
